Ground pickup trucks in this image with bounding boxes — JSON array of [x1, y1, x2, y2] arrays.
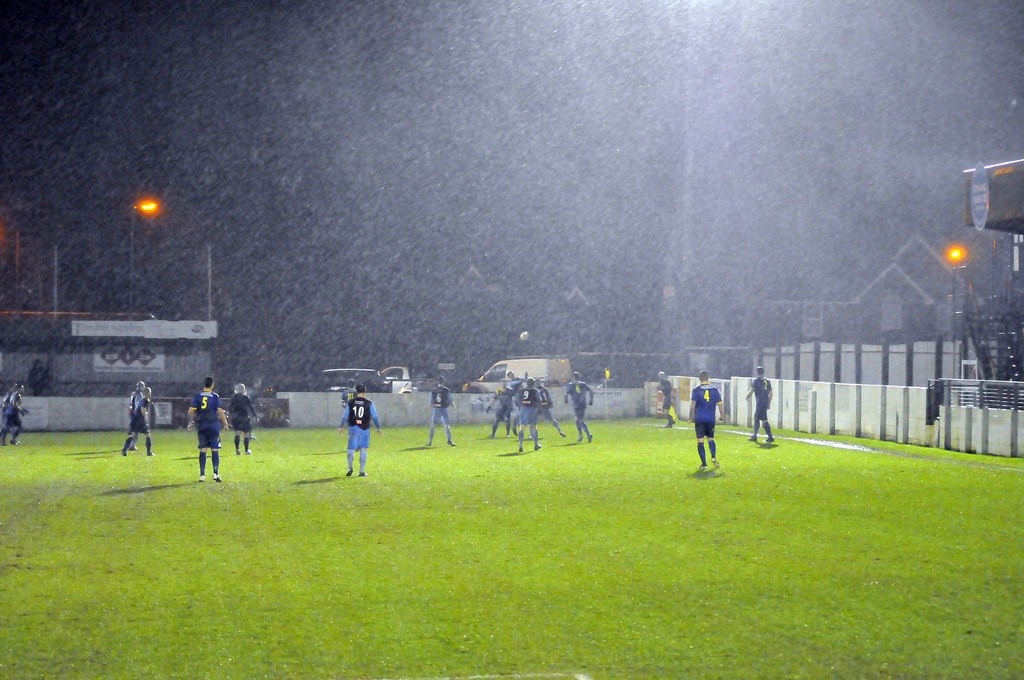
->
[[377, 366, 436, 394]]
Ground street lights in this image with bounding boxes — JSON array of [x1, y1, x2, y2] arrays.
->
[[948, 245, 964, 379], [128, 198, 159, 310]]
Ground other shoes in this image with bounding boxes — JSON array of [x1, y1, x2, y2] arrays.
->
[[766, 437, 774, 443], [213, 473, 221, 482], [559, 432, 566, 438], [512, 427, 517, 435], [245, 449, 252, 454], [147, 451, 155, 456], [346, 468, 353, 476], [747, 435, 757, 441], [359, 472, 368, 476], [428, 443, 431, 446], [507, 434, 510, 438], [488, 434, 495, 439], [712, 456, 719, 469], [528, 435, 534, 440], [665, 420, 675, 428], [2, 438, 20, 446], [122, 448, 127, 456], [447, 440, 455, 446], [577, 436, 583, 440], [588, 433, 592, 442], [535, 444, 542, 450], [199, 475, 205, 481], [698, 463, 707, 469], [128, 446, 139, 451], [519, 447, 523, 452], [236, 448, 240, 456]]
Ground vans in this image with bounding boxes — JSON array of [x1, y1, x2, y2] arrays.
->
[[462, 354, 574, 394]]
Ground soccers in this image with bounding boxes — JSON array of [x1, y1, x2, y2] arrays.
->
[[520, 332, 529, 340]]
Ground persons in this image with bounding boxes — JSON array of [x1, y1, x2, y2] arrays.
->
[[29, 360, 46, 395], [339, 385, 382, 476], [227, 379, 261, 455], [187, 377, 228, 482], [0, 384, 29, 445], [340, 380, 357, 409], [564, 372, 593, 442], [658, 372, 675, 428], [122, 382, 155, 456], [427, 376, 456, 446], [746, 366, 774, 442], [486, 371, 566, 452], [688, 370, 724, 469]]
